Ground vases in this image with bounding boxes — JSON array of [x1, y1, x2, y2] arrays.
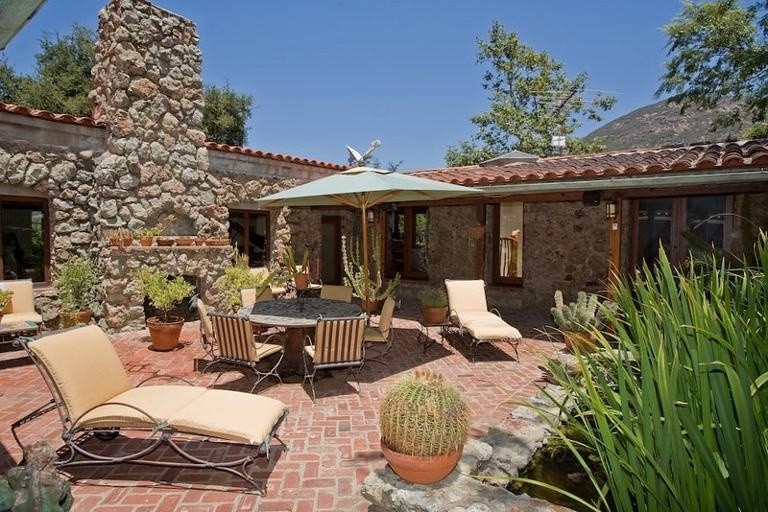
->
[[110, 237, 133, 246], [194, 238, 204, 246], [156, 240, 174, 246], [175, 238, 194, 245], [205, 239, 230, 245]]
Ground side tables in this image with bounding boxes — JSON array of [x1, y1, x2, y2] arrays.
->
[[416, 319, 456, 355]]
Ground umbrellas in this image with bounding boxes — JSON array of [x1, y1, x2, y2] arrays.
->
[[250, 163, 485, 324]]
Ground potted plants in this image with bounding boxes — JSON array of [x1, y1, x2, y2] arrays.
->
[[136, 269, 197, 350], [0, 288, 14, 324], [417, 288, 449, 324], [377, 371, 470, 484], [140, 228, 158, 246], [340, 230, 400, 315], [283, 245, 314, 290], [53, 257, 100, 328], [548, 289, 618, 355]]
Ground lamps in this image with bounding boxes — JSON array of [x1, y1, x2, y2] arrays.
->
[[604, 196, 619, 226], [367, 207, 378, 223]]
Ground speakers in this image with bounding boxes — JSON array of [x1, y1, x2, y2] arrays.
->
[[582, 191, 600, 207]]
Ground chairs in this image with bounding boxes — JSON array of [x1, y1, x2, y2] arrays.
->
[[320, 284, 354, 304], [0, 279, 43, 343], [196, 299, 219, 371], [302, 312, 367, 403], [444, 279, 521, 362], [240, 286, 279, 341], [363, 297, 396, 360], [11, 324, 289, 497], [207, 312, 285, 395]]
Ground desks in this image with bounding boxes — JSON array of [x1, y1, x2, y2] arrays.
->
[[237, 298, 362, 376], [0, 320, 40, 364]]
